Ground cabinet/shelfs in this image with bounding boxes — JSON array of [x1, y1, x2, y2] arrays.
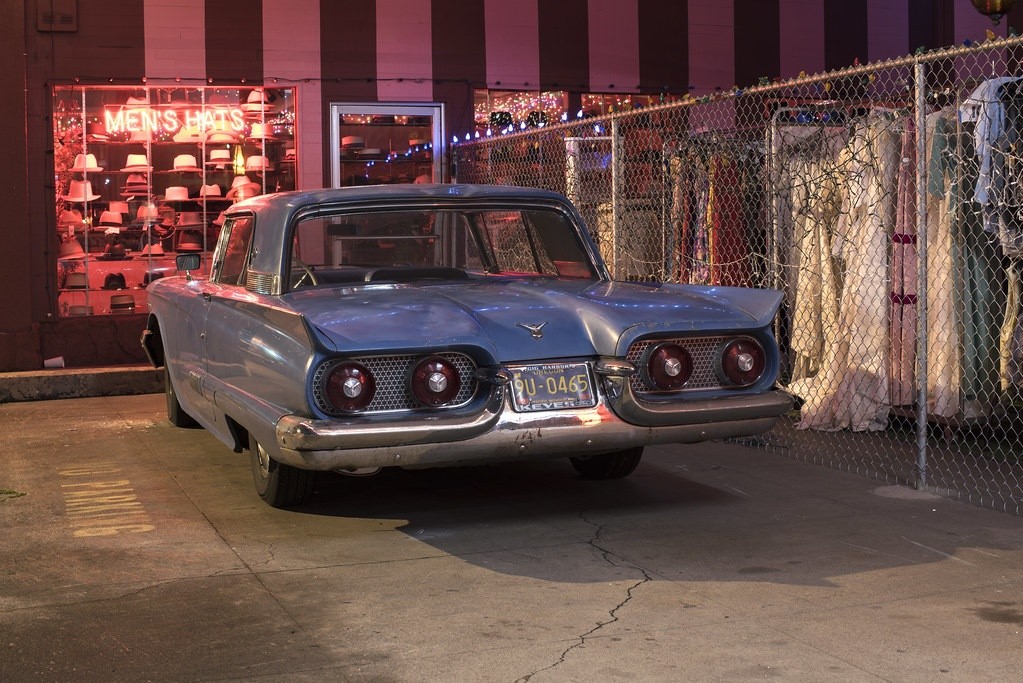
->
[[330, 101, 447, 266], [54, 86, 298, 317]]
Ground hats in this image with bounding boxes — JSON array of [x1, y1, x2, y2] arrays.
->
[[53, 88, 296, 317], [490, 111, 513, 125], [527, 111, 549, 126], [341, 135, 365, 149]]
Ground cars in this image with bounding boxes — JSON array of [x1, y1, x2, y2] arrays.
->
[[140, 184, 805, 506]]
